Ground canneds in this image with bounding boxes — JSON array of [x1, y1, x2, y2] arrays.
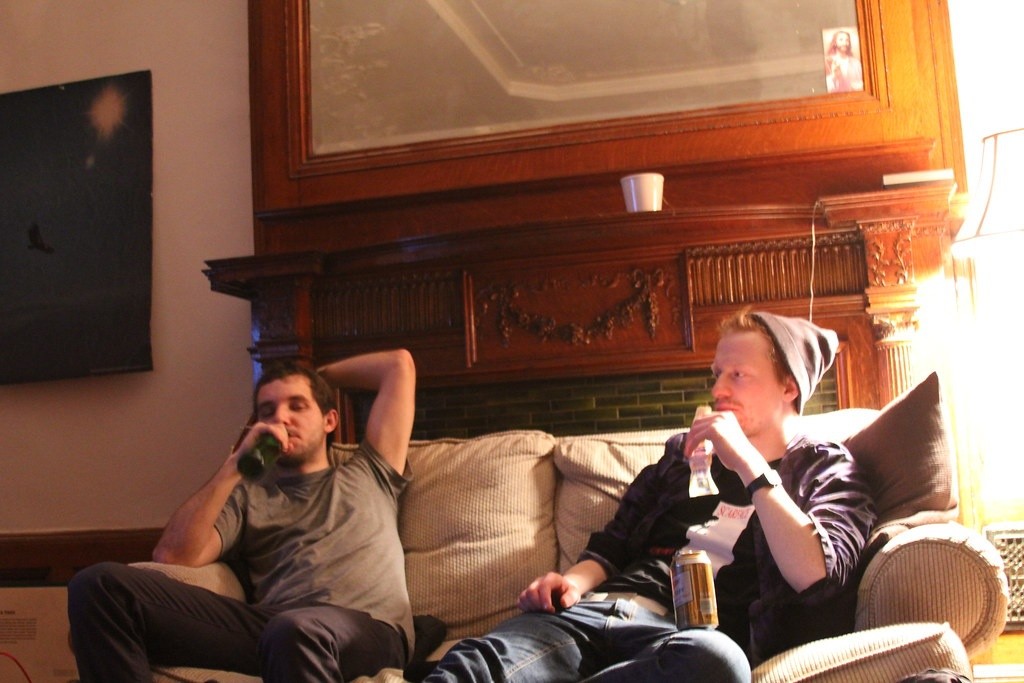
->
[[670, 550, 718, 630]]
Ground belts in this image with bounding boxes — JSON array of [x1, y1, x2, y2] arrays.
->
[[588, 592, 669, 617]]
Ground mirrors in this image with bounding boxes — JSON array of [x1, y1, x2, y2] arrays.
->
[[282, 0, 887, 180]]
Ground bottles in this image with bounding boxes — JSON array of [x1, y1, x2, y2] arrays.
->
[[237, 433, 280, 480]]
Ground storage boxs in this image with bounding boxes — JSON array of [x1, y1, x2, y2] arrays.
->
[[0, 585, 80, 683]]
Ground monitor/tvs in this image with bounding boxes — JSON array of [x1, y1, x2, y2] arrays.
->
[[0, 70, 153, 385]]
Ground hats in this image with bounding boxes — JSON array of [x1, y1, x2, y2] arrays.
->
[[749, 311, 839, 416]]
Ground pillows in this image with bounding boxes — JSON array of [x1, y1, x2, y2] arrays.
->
[[840, 371, 961, 559]]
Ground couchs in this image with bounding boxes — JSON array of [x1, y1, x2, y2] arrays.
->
[[128, 406, 1007, 683]]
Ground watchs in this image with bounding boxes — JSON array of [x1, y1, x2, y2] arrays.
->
[[741, 470, 782, 504]]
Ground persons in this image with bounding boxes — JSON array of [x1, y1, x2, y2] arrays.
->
[[422, 305, 879, 683], [826, 31, 864, 93], [67, 349, 415, 683]]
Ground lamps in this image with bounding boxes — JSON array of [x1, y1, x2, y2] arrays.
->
[[950, 129, 1024, 258]]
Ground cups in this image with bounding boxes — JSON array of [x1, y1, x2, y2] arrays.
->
[[620, 172, 665, 213]]
